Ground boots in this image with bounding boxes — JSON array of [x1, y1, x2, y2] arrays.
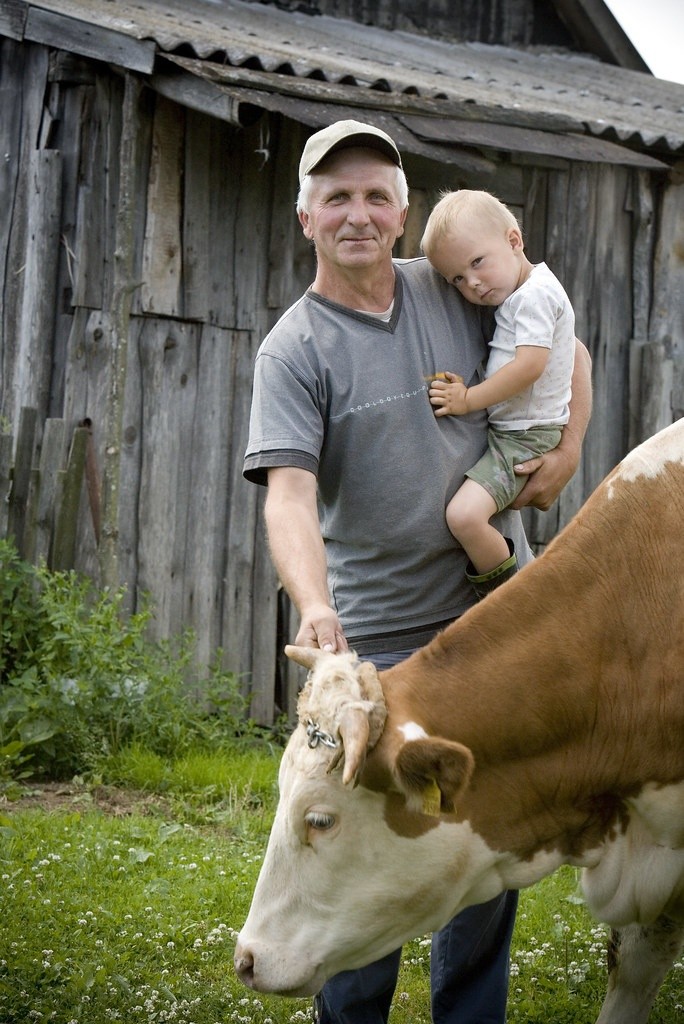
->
[[463, 540, 520, 600]]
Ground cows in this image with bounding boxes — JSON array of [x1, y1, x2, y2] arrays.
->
[[232, 411, 683, 1024]]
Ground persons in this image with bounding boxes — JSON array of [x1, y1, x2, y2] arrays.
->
[[242, 119, 592, 1024], [421, 190, 576, 602]]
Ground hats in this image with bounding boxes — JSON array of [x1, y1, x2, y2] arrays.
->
[[296, 120, 402, 187]]
